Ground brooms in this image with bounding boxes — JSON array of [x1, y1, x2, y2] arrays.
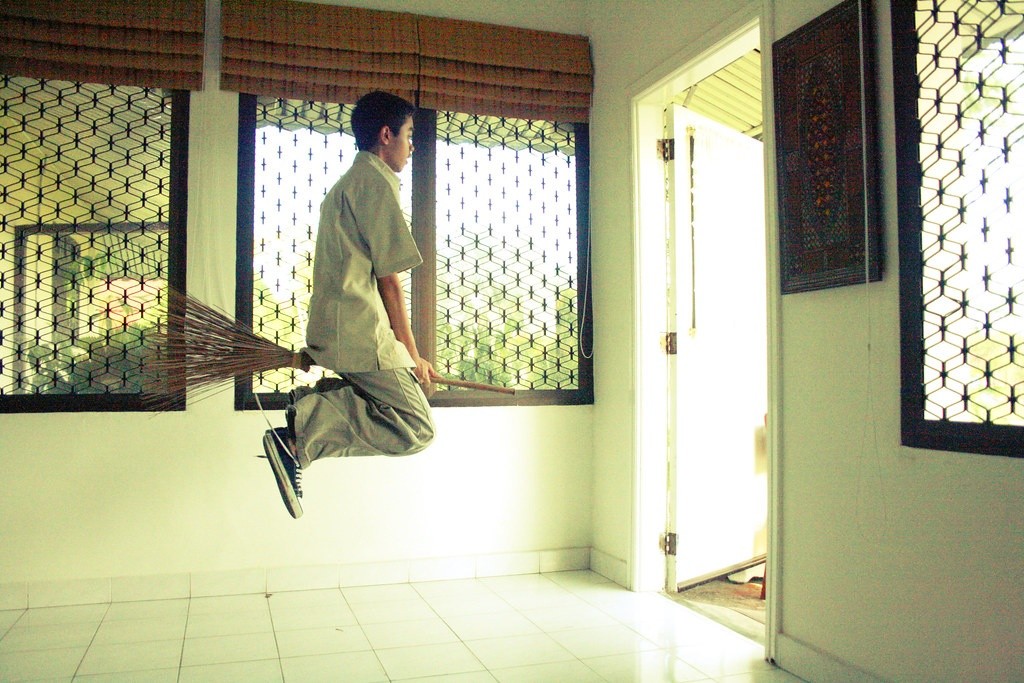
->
[[141, 286, 518, 418]]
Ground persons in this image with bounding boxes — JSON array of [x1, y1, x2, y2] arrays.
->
[[262, 92, 443, 519]]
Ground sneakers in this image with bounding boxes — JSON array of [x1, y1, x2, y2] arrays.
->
[[262, 427, 304, 519]]
